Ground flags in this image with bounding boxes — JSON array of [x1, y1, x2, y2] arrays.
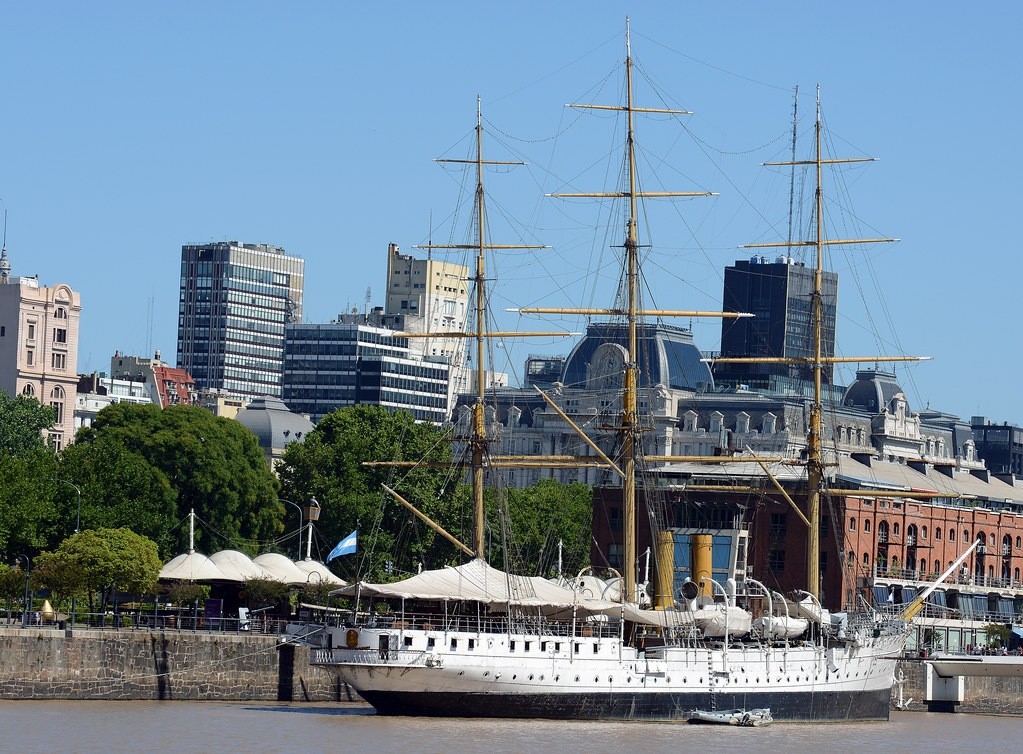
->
[[326, 531, 356, 565]]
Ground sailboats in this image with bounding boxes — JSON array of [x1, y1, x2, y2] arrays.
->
[[307, 13, 911, 725]]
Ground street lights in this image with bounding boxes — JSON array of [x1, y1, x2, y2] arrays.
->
[[13, 554, 29, 628]]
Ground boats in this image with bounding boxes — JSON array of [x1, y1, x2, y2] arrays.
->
[[689, 704, 774, 728]]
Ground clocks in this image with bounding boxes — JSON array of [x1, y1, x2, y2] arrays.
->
[[606, 359, 617, 368]]
